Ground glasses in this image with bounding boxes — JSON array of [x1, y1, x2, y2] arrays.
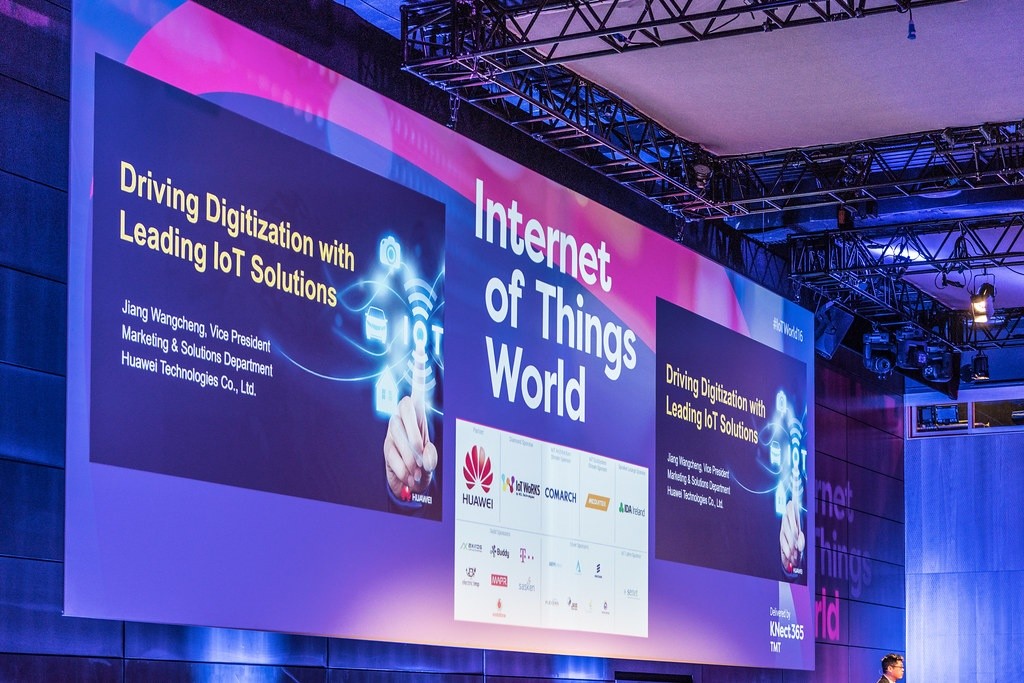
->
[[891, 666, 905, 671]]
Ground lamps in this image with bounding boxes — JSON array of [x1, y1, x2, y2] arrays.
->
[[979, 122, 994, 142], [862, 322, 954, 382], [941, 127, 956, 145], [690, 163, 712, 189], [814, 301, 854, 361], [970, 267, 997, 323], [974, 349, 989, 379]]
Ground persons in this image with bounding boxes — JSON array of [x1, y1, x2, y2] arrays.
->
[[876, 653, 905, 683], [384, 340, 438, 515], [780, 462, 805, 582]]
